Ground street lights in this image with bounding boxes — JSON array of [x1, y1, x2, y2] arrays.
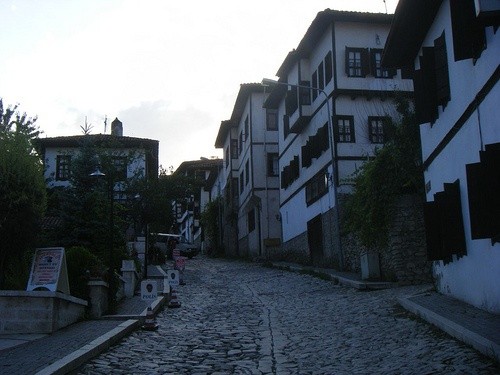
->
[[90, 162, 113, 314], [261, 75, 344, 271], [133, 193, 148, 280], [199, 157, 223, 255]]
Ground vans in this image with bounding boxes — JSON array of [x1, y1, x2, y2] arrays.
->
[[149, 233, 199, 260]]
